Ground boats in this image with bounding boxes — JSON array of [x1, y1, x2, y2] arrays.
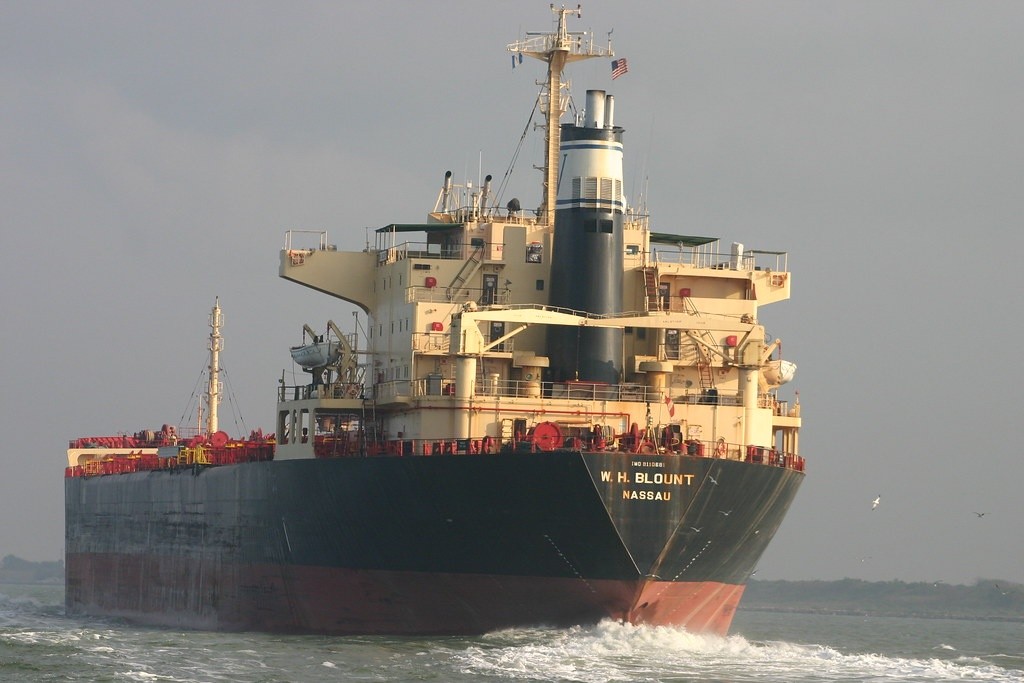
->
[[63, 2, 815, 643]]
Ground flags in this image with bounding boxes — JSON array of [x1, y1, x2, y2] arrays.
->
[[511, 53, 523, 69], [611, 57, 628, 80]]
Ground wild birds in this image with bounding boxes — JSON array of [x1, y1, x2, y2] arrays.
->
[[645, 574, 663, 580], [750, 571, 756, 577], [719, 510, 733, 516], [690, 526, 705, 533], [932, 579, 944, 587], [993, 584, 1015, 598], [755, 530, 760, 535], [973, 512, 990, 521], [872, 495, 881, 511], [708, 474, 721, 487]]
